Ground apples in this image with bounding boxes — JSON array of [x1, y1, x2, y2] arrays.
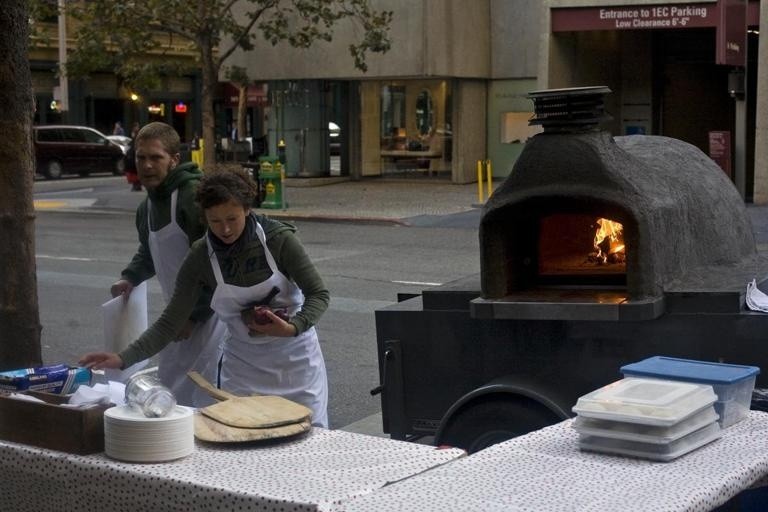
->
[[255, 307, 273, 325], [271, 310, 289, 323]]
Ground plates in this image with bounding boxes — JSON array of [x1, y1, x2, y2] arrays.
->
[[103, 400, 196, 463]]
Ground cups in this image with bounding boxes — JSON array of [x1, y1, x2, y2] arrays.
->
[[123, 372, 176, 419]]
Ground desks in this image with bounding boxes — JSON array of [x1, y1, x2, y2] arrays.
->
[[0, 401, 768, 512], [379, 150, 442, 179]]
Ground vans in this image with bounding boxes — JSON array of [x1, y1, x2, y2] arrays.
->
[[31, 125, 125, 179]]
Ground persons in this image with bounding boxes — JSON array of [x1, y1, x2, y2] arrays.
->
[[112, 122, 125, 135], [108, 121, 233, 406], [130, 122, 141, 139], [76, 159, 334, 432]]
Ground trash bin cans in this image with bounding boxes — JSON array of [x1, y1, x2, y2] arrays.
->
[[259, 156, 288, 210]]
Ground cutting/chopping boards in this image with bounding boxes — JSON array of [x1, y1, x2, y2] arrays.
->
[[101, 281, 150, 383], [185, 369, 313, 445]]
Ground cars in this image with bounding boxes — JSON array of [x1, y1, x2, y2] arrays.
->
[[109, 133, 132, 151]]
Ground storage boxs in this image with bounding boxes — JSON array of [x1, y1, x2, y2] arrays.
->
[[619, 355, 761, 429]]
